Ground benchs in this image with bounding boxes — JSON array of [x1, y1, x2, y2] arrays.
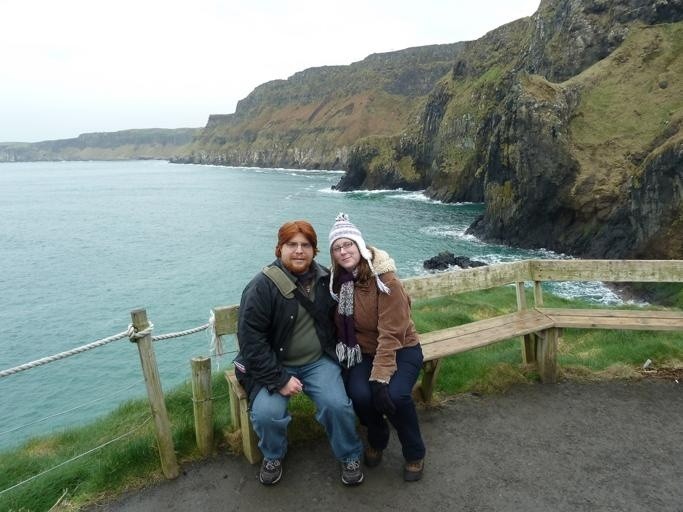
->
[[534, 259, 683, 384], [213, 261, 554, 465]]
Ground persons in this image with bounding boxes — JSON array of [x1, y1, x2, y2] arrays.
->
[[328, 211, 427, 484], [228, 220, 366, 487]]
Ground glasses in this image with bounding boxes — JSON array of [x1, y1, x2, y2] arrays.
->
[[332, 242, 354, 252], [287, 241, 311, 249]]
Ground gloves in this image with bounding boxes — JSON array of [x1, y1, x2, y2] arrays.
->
[[372, 382, 396, 416]]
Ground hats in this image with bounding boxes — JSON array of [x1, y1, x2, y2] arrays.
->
[[328, 212, 372, 261]]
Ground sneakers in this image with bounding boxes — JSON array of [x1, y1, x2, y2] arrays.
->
[[366, 443, 382, 464], [341, 459, 364, 485], [259, 457, 283, 485], [403, 460, 424, 481]]
